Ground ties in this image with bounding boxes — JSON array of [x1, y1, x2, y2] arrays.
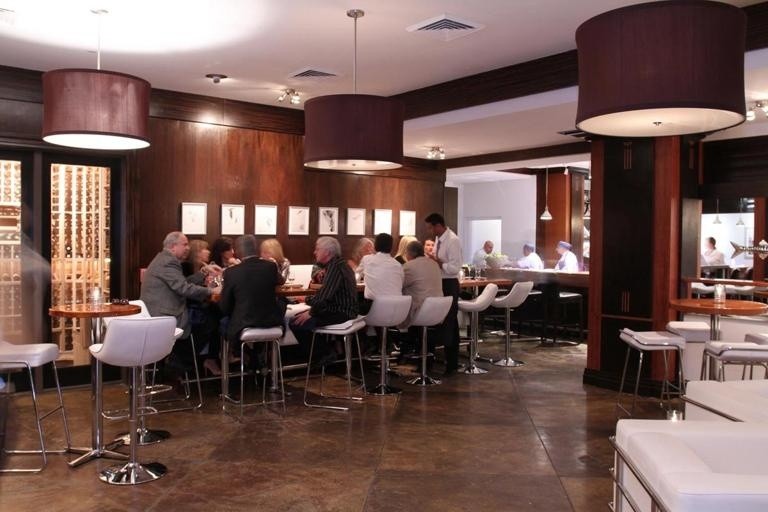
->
[[435, 240, 440, 258]]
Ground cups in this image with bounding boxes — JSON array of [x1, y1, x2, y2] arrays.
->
[[713, 284, 728, 304], [214, 275, 224, 285], [474, 268, 481, 279], [457, 271, 465, 282]]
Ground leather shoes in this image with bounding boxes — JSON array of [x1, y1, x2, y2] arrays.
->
[[443, 369, 456, 377], [410, 366, 430, 372]]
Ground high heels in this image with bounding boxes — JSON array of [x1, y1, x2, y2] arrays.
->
[[204, 360, 221, 376], [218, 350, 240, 363]]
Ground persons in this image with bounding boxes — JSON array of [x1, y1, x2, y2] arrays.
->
[[472, 240, 495, 269], [552, 240, 580, 273], [181, 238, 241, 377], [208, 234, 446, 379], [702, 236, 726, 266], [140, 231, 222, 373], [510, 243, 543, 270], [410, 211, 461, 379]]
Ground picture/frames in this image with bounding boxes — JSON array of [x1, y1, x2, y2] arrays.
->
[[181, 202, 416, 236]]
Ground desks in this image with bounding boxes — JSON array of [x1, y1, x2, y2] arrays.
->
[[701, 264, 729, 278]]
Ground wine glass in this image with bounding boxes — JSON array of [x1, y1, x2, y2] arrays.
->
[[286, 268, 296, 284]]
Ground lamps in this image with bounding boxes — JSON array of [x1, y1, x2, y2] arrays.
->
[[736, 197, 744, 225], [713, 198, 722, 224], [540, 168, 553, 221]]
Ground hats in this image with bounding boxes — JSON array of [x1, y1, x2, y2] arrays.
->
[[559, 241, 572, 251], [525, 243, 534, 250]]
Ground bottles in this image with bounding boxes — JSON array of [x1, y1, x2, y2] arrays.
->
[[462, 265, 471, 276]]
